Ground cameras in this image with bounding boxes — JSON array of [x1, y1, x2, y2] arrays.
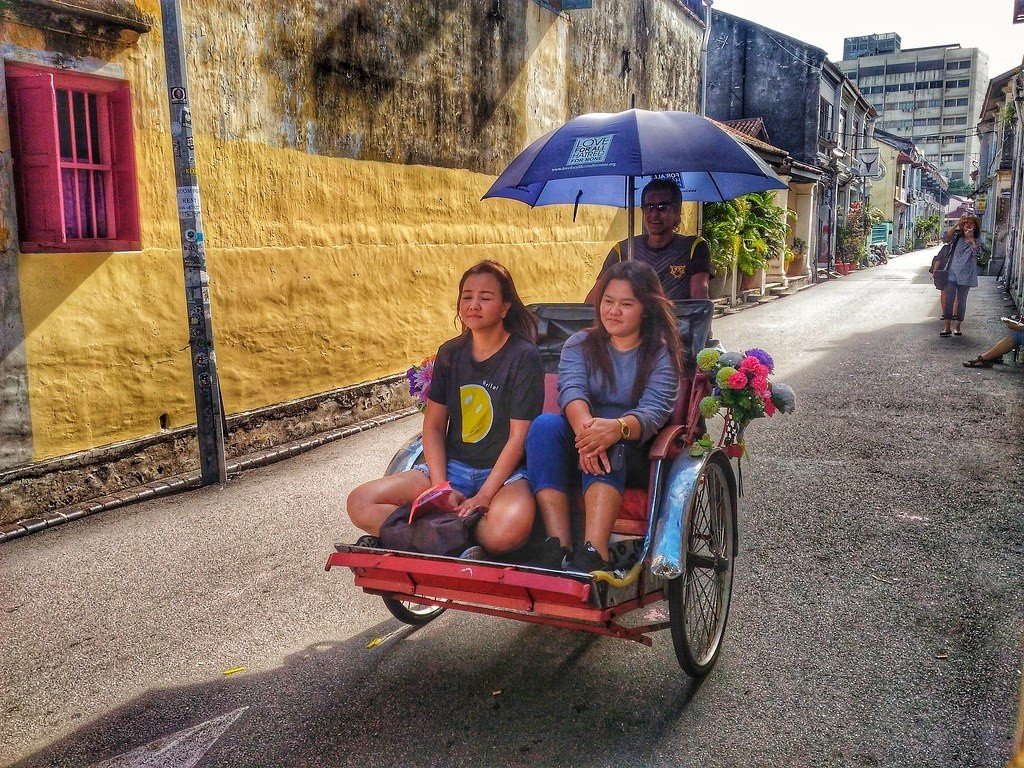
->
[[962, 210, 967, 217]]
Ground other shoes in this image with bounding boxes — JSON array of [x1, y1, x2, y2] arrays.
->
[[940, 330, 952, 334], [954, 329, 962, 334], [356, 535, 381, 549], [952, 316, 957, 319], [448, 537, 485, 561], [940, 315, 945, 320]]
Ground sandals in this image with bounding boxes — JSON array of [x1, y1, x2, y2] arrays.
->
[[963, 355, 993, 368], [993, 355, 1004, 364]]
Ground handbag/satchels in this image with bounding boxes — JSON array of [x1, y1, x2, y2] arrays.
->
[[377, 503, 485, 556], [934, 270, 948, 289]]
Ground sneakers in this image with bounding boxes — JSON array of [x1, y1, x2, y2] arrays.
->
[[567, 540, 610, 584], [528, 536, 573, 577]]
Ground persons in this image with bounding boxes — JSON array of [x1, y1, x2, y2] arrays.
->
[[521, 259, 684, 601], [929, 231, 958, 321], [345, 260, 546, 561], [962, 317, 1024, 368], [940, 214, 986, 339], [584, 179, 728, 446]]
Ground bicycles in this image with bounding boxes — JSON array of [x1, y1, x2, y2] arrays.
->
[[865, 244, 888, 265]]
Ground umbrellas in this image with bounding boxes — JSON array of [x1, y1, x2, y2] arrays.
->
[[481, 94, 792, 260]]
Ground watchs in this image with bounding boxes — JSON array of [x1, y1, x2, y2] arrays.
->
[[616, 417, 630, 440]]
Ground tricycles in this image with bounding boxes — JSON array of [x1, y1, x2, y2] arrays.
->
[[325, 268, 740, 680]]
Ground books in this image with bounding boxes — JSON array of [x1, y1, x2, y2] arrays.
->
[[1001, 317, 1024, 326]]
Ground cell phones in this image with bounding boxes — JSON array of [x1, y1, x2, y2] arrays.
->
[[577, 443, 625, 471]]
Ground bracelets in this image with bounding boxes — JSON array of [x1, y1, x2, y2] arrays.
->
[[971, 239, 976, 244]]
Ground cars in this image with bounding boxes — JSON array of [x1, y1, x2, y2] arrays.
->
[[942, 231, 952, 243]]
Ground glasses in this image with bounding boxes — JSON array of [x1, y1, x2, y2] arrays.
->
[[641, 202, 679, 212]]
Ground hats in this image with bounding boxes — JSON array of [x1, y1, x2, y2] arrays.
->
[[959, 214, 981, 230]]
